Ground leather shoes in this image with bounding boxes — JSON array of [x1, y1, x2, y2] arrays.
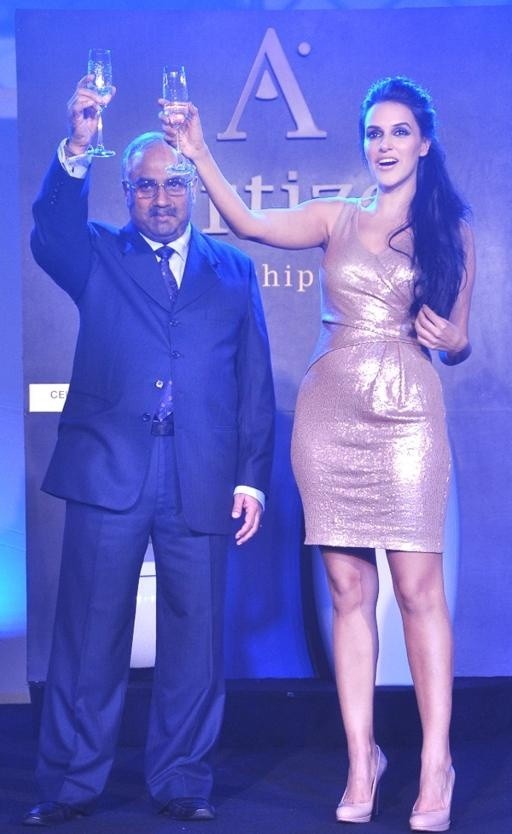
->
[[163, 796, 215, 822], [19, 800, 81, 828]]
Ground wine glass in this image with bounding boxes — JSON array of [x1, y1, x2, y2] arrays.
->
[[162, 64, 196, 175], [85, 46, 117, 159]]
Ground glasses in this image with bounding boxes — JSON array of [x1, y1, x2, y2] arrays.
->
[[124, 178, 193, 198]]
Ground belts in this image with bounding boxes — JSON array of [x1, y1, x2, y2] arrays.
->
[[148, 418, 175, 436]]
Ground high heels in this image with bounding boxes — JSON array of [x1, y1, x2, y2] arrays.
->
[[333, 745, 388, 824], [408, 762, 456, 832]]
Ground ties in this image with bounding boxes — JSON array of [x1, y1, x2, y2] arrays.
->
[[152, 245, 181, 304]]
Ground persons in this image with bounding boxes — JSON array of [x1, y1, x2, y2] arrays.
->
[[144, 73, 480, 834], [17, 72, 279, 831]]
